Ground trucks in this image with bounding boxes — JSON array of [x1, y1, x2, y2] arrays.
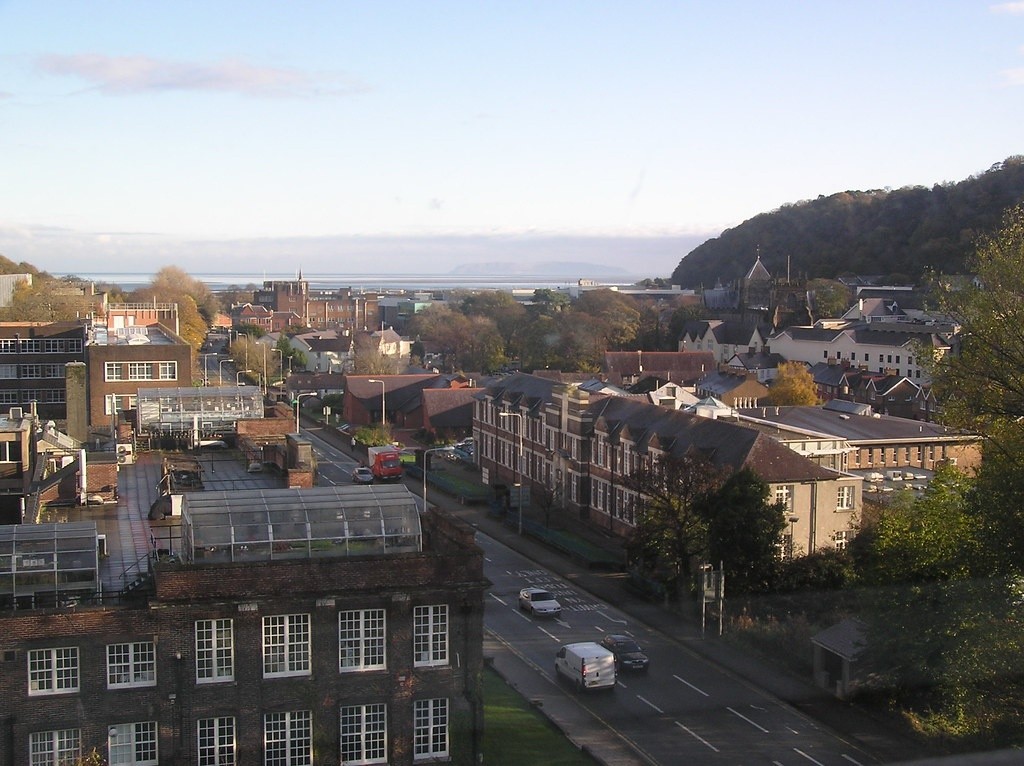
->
[[395, 434, 479, 461], [368, 446, 406, 483]]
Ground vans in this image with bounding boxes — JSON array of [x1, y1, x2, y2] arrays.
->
[[555, 643, 619, 692]]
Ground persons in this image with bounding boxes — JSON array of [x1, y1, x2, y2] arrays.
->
[[351, 437, 356, 452]]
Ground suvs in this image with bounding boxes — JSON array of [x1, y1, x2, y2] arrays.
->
[[600, 633, 649, 676]]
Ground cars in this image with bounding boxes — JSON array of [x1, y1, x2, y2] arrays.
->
[[248, 463, 262, 473], [519, 587, 562, 618], [861, 470, 928, 494], [353, 467, 374, 484]]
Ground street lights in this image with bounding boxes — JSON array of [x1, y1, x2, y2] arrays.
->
[[204, 352, 217, 387], [238, 333, 249, 374], [296, 392, 318, 434], [220, 359, 234, 386], [369, 379, 391, 425], [499, 412, 536, 535], [255, 342, 267, 395], [271, 349, 283, 402], [423, 446, 456, 514], [236, 370, 253, 388]]
